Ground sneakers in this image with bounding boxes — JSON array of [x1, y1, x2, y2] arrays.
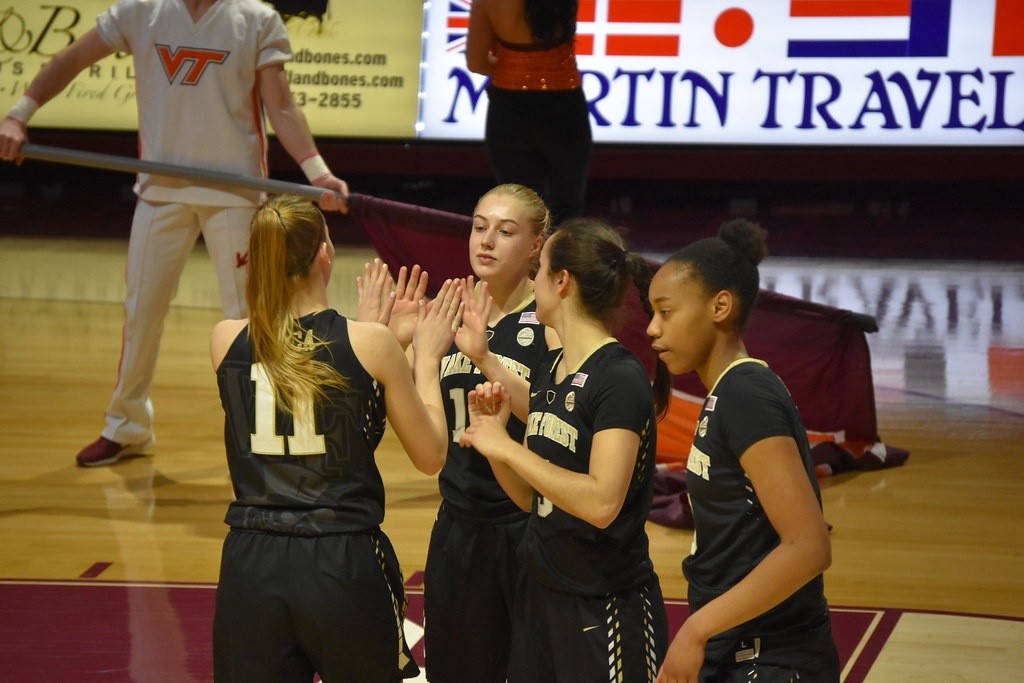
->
[[74, 436, 158, 468]]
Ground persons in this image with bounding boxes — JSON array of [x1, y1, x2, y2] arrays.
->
[[459, 217, 670, 683], [646, 217, 839, 683], [466, 0, 592, 226], [209, 193, 463, 683], [384, 182, 564, 683]]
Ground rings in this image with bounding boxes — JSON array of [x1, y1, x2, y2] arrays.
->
[[452, 320, 459, 332]]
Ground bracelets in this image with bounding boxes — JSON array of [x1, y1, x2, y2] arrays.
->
[[0, 0, 348, 466], [6, 94, 40, 124], [299, 151, 331, 182]]
